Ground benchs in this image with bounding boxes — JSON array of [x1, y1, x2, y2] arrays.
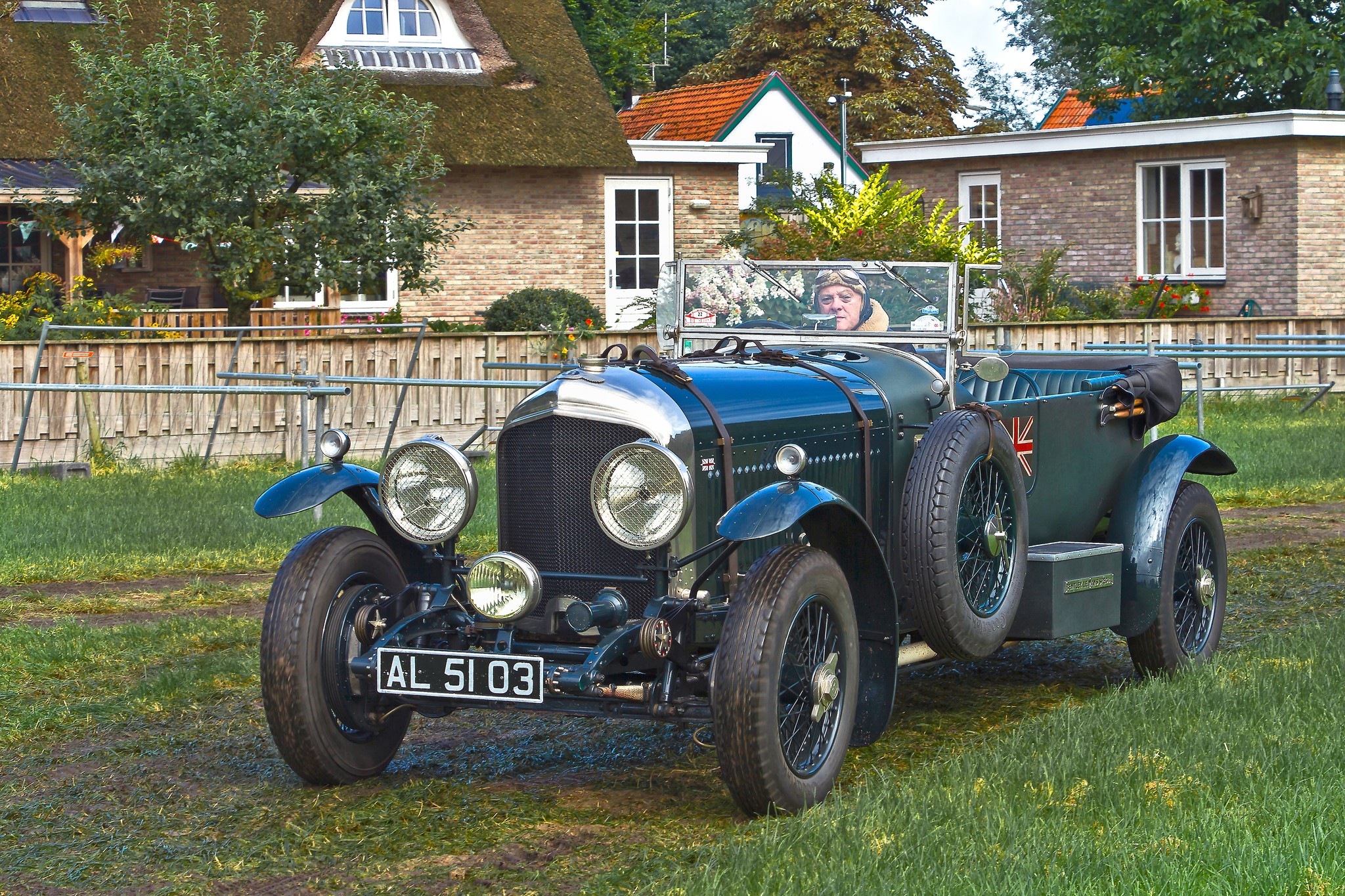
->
[[920, 360, 1129, 405]]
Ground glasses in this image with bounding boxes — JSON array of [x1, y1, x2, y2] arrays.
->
[[814, 267, 868, 292]]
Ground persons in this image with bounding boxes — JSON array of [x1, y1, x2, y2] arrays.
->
[[802, 269, 901, 335], [1172, 233, 1197, 271]]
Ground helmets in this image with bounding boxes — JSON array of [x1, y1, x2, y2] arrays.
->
[[813, 258, 870, 323]]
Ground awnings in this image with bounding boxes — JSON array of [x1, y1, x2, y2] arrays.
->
[[0, 154, 357, 205], [9, 0, 111, 25]]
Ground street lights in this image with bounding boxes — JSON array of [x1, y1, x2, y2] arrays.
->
[[828, 77, 854, 194]]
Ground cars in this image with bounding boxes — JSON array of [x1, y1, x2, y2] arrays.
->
[[255, 255, 1238, 820]]
